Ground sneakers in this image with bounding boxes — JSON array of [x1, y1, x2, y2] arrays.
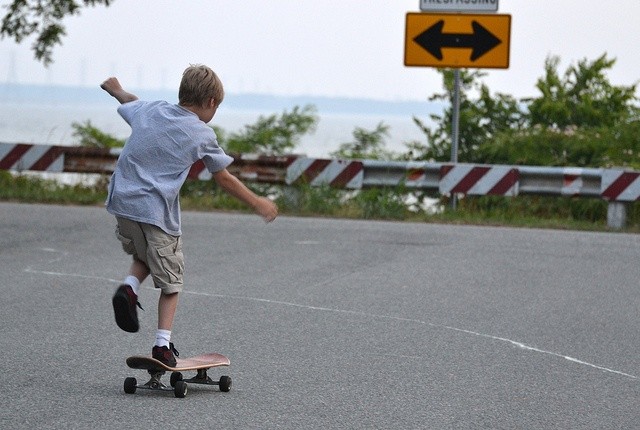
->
[[112, 284, 144, 333], [152, 343, 179, 367]]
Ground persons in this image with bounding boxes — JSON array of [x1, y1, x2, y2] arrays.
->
[[99, 63, 279, 367]]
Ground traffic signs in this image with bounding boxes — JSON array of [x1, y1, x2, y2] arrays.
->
[[419, 0, 498, 13]]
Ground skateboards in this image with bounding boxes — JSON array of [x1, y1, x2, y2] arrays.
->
[[123, 353, 232, 398]]
